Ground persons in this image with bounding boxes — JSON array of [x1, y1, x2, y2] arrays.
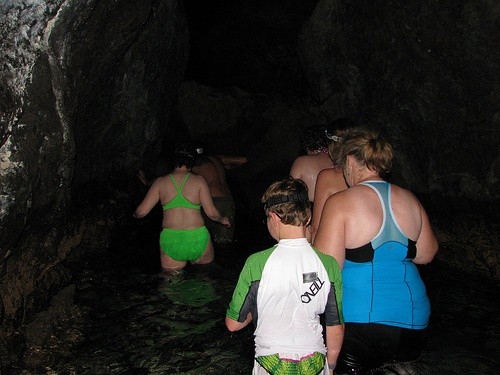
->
[[132, 138, 248, 273], [225, 177, 345, 375], [289, 120, 440, 375]]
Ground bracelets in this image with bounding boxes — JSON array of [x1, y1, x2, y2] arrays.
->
[[328, 364, 337, 369]]
